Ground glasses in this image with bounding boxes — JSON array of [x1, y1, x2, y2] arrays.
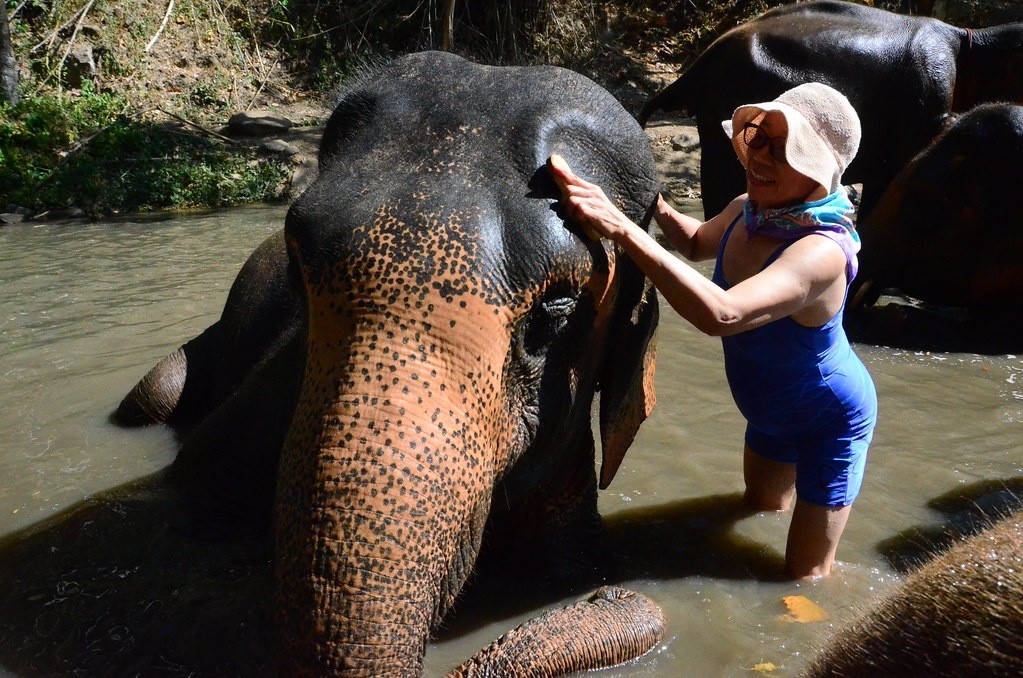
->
[[742, 122, 788, 164]]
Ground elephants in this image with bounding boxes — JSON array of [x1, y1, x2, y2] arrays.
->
[[633, 0, 1023, 355], [103, 46, 667, 678]]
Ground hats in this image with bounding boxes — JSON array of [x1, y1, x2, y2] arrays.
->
[[731, 81, 862, 204]]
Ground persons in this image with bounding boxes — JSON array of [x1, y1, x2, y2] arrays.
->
[[547, 81, 877, 576]]
